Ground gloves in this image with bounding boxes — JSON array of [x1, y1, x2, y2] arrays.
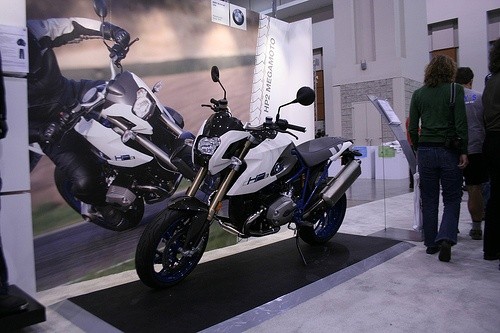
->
[[100, 22, 130, 49]]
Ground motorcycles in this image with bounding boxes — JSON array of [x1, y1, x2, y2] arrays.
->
[[30, 0, 226, 232], [134, 65, 362, 291]]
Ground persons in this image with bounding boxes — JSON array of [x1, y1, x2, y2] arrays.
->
[[405, 45, 500, 262], [25, 17, 130, 228]]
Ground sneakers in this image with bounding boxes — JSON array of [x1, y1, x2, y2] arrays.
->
[[103, 203, 126, 228]]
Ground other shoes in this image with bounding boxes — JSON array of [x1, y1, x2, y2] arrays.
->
[[409, 188, 414, 192], [426, 245, 439, 254], [484, 252, 500, 260], [469, 228, 482, 240], [438, 242, 450, 262]]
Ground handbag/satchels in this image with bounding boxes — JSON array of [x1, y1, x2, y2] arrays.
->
[[412, 164, 423, 232], [465, 153, 489, 185]]
